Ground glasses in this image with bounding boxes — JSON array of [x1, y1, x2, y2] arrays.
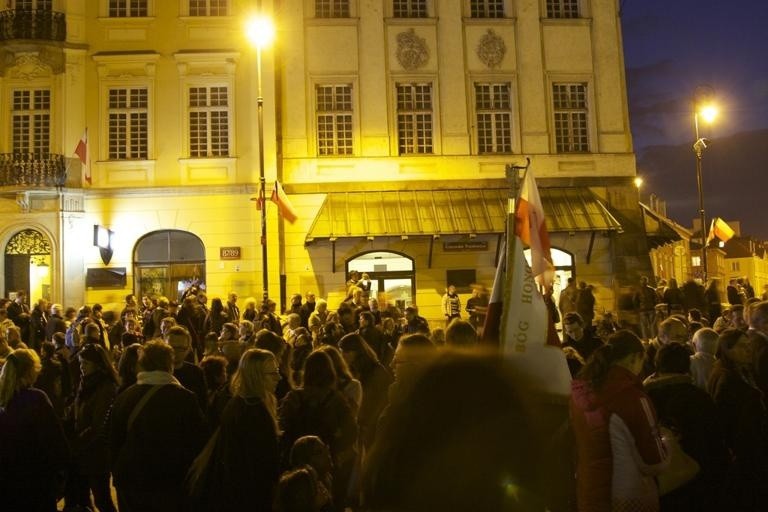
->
[[169, 343, 189, 353], [84, 343, 97, 349]]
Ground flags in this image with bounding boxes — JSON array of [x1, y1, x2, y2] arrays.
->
[[484, 199, 576, 412], [512, 157, 556, 297], [253, 177, 264, 215], [74, 126, 93, 189], [714, 216, 736, 244], [269, 180, 296, 226], [705, 218, 715, 247]]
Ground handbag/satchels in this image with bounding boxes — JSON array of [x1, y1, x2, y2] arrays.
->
[[177, 431, 220, 505]]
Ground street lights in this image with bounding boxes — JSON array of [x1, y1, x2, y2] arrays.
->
[[692, 83, 721, 289], [243, 13, 276, 303]]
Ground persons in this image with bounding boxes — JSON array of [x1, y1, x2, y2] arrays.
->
[[1, 269, 767, 512]]
